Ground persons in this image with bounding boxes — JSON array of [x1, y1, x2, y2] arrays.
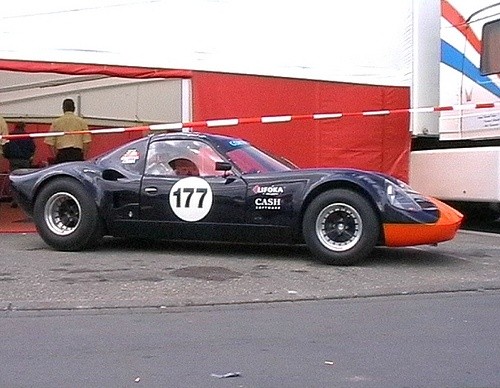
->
[[2, 122, 37, 173], [44, 99, 92, 164], [0, 113, 9, 157]]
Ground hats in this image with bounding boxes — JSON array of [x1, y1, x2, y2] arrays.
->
[[16, 121, 26, 128]]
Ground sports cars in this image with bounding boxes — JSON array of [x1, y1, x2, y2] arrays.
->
[[8, 132, 466, 265]]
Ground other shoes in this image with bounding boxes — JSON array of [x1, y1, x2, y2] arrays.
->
[[11, 202, 17, 208]]
